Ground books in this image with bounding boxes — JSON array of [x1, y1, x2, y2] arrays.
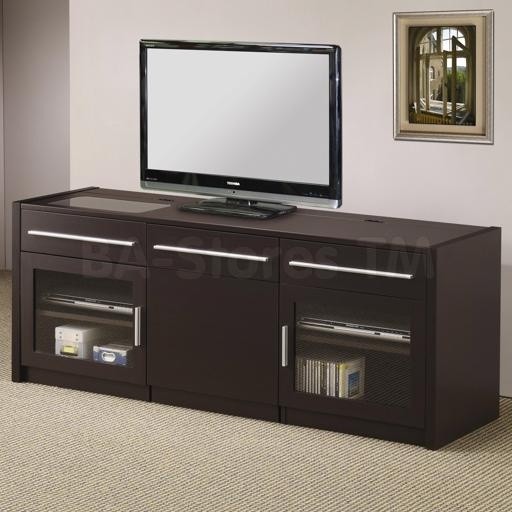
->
[[295, 347, 365, 400]]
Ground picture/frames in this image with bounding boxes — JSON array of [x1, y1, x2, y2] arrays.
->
[[392, 8, 495, 145]]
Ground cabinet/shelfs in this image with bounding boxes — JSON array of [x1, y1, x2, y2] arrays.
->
[[12, 185, 501, 452]]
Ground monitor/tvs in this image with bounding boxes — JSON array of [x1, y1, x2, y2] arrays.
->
[[139, 38, 343, 220]]
